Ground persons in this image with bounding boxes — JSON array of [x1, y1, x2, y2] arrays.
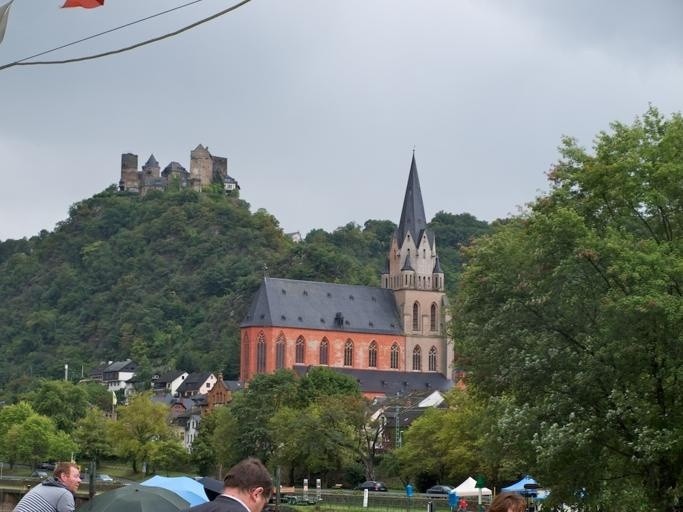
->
[[180, 456, 276, 512], [455, 498, 468, 512], [11, 462, 82, 512], [481, 493, 526, 512]]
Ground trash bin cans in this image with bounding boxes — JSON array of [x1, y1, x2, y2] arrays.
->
[[407, 485, 413, 497]]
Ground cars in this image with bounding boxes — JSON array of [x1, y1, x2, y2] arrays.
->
[[574, 488, 590, 498], [425, 484, 455, 499], [31, 462, 113, 481], [358, 479, 388, 492]]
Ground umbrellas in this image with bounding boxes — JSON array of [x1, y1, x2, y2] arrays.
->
[[194, 475, 224, 501], [74, 485, 192, 512], [138, 475, 210, 508]]
